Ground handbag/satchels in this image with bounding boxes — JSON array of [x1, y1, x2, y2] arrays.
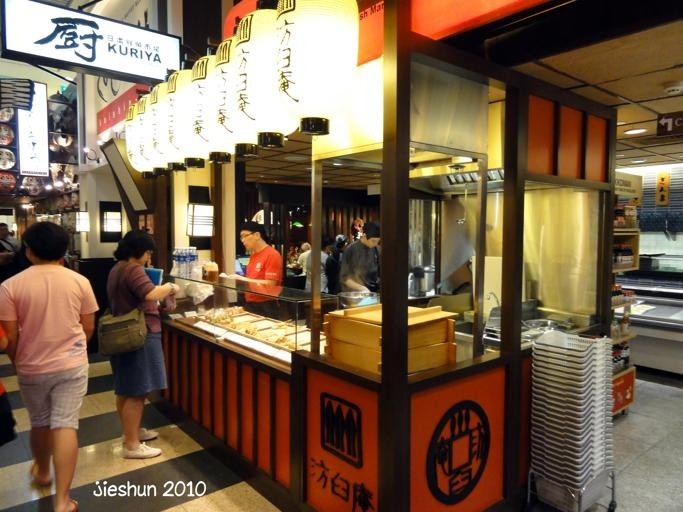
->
[[95, 309, 146, 356]]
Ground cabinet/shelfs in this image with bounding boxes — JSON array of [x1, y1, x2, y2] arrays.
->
[[162, 269, 339, 502]]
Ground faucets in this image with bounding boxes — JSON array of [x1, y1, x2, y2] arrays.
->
[[487, 292, 501, 308]]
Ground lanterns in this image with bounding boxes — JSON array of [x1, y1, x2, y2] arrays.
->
[[124, 2, 358, 180]]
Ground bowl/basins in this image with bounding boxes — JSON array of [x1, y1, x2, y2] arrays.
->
[[336, 291, 377, 309]]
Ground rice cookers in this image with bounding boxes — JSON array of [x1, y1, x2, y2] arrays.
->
[[412, 264, 436, 296]]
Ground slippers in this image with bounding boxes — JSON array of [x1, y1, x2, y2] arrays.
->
[[28, 460, 78, 512]]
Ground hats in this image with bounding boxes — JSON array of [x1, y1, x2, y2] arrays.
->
[[362, 223, 379, 238]]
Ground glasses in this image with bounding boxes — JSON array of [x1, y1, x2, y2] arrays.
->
[[239, 232, 257, 241]]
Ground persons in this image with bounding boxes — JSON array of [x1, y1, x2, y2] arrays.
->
[[291, 218, 381, 328], [226, 222, 283, 319], [98, 229, 180, 458], [0, 223, 16, 252], [0, 221, 100, 511], [0, 327, 18, 446]]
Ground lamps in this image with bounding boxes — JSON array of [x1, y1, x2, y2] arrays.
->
[[124, 0, 359, 181]]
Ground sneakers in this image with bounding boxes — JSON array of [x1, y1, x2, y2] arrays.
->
[[119, 446, 162, 459], [120, 428, 159, 443]]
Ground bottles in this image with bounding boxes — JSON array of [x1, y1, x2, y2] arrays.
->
[[611, 199, 635, 376], [171, 246, 199, 280]]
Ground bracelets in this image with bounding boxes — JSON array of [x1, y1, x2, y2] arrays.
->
[[168, 282, 173, 296]]
